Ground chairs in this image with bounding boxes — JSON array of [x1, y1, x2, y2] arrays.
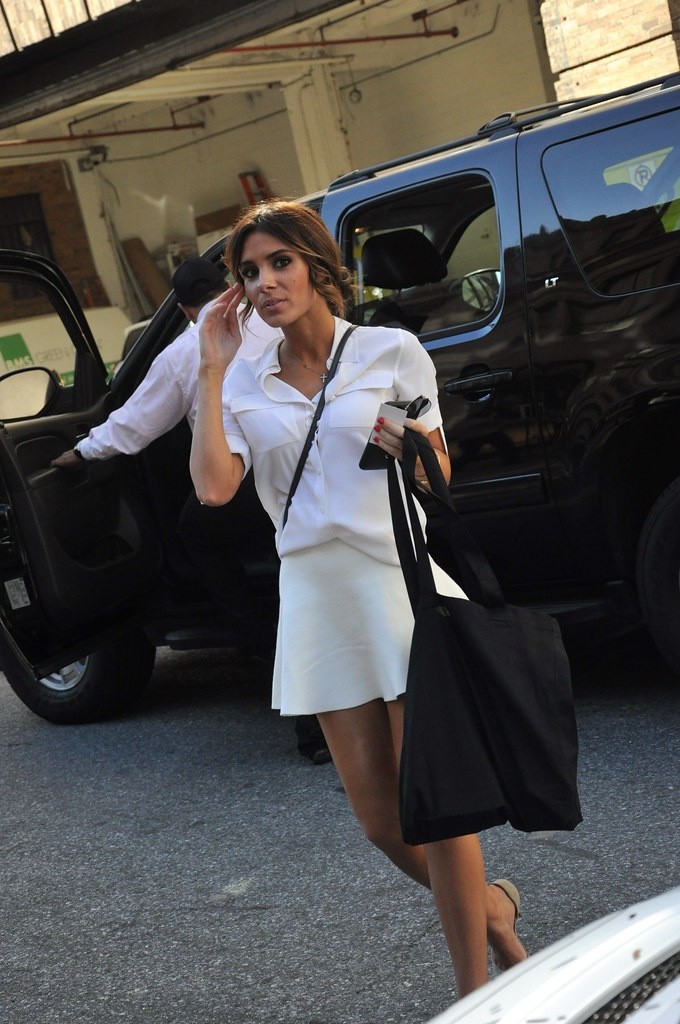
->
[[362, 228, 448, 289], [591, 183, 667, 253]]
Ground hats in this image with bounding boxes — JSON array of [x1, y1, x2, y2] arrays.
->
[[173, 259, 224, 301]]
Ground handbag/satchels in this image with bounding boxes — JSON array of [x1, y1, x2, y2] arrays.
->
[[386, 425, 582, 846]]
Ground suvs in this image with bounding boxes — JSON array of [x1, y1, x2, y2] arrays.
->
[[0, 74, 680, 723]]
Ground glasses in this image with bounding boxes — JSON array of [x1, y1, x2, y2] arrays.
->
[[404, 395, 431, 420]]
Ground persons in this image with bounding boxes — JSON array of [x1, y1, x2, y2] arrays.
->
[[49, 257, 331, 765], [188, 203, 528, 998]]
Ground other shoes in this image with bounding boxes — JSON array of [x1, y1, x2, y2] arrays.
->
[[298, 743, 331, 764], [488, 878, 530, 978]]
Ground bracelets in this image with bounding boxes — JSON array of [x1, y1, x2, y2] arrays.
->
[[73, 443, 83, 459], [421, 450, 440, 484]]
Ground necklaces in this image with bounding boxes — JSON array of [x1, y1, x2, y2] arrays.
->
[[283, 342, 327, 382]]
